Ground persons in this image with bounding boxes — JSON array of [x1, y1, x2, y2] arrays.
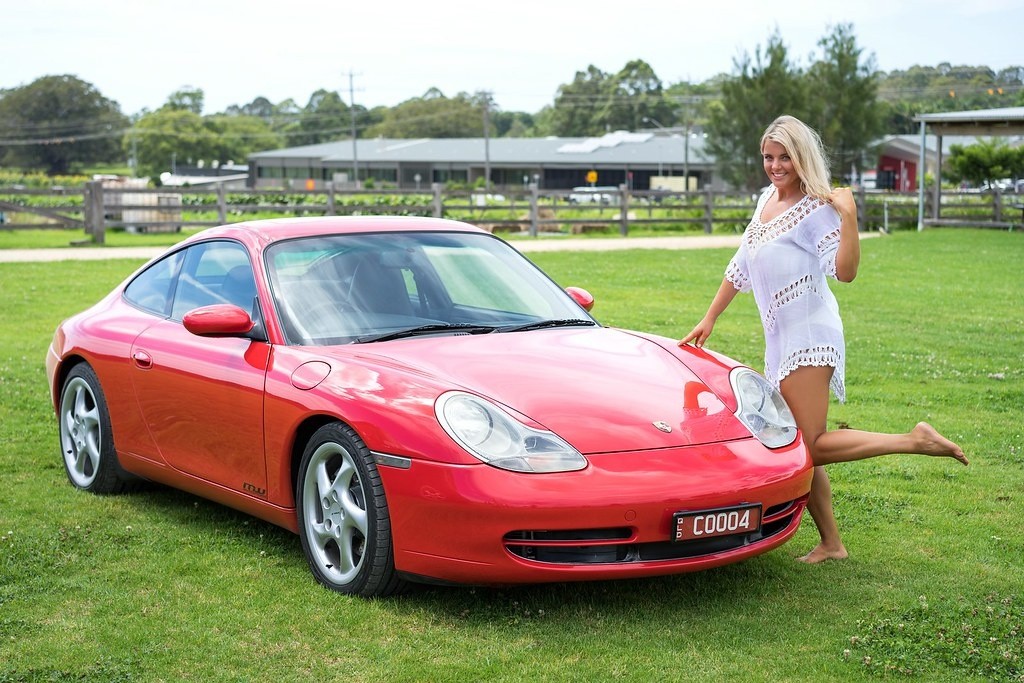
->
[[675, 115, 969, 564]]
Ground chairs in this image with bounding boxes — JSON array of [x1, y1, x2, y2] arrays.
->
[[351, 254, 417, 317], [217, 265, 257, 319]]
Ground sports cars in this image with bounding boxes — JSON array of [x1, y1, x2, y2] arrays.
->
[[45, 214, 817, 598]]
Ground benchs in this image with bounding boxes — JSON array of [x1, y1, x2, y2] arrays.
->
[[158, 279, 349, 322]]
